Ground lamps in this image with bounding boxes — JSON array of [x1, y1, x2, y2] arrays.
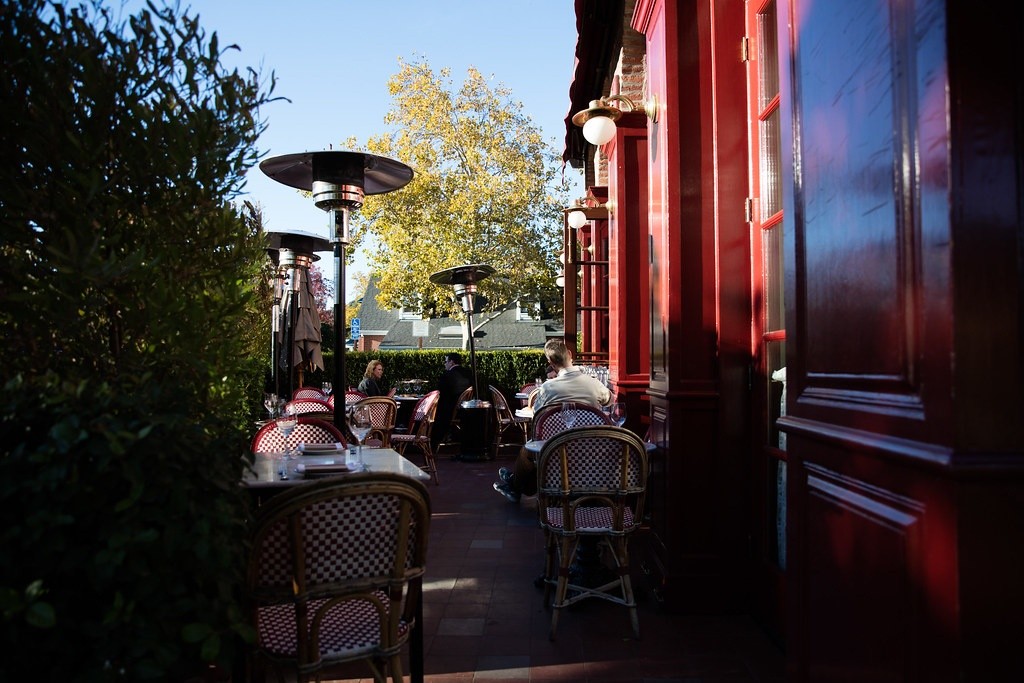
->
[[556, 241, 593, 265], [571, 94, 659, 146], [554, 267, 582, 288], [563, 191, 615, 228]]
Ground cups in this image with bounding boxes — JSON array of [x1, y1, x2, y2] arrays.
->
[[609, 403, 627, 428]]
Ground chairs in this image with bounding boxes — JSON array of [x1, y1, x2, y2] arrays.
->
[[239, 386, 649, 683]]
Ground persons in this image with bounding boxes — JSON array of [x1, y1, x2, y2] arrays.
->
[[357, 359, 390, 397], [431, 351, 473, 450], [492, 337, 617, 502]]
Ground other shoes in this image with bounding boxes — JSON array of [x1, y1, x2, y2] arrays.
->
[[498, 467, 523, 494], [493, 481, 519, 503]]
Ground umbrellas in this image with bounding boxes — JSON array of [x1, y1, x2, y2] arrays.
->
[[277, 267, 325, 390]]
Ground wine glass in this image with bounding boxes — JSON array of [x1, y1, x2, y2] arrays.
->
[[560, 402, 576, 429], [349, 404, 372, 468], [264, 393, 298, 458], [322, 381, 332, 402], [403, 377, 422, 398]]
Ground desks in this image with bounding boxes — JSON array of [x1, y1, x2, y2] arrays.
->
[[393, 394, 424, 404], [240, 448, 431, 485], [515, 393, 528, 400], [524, 439, 657, 454]]
